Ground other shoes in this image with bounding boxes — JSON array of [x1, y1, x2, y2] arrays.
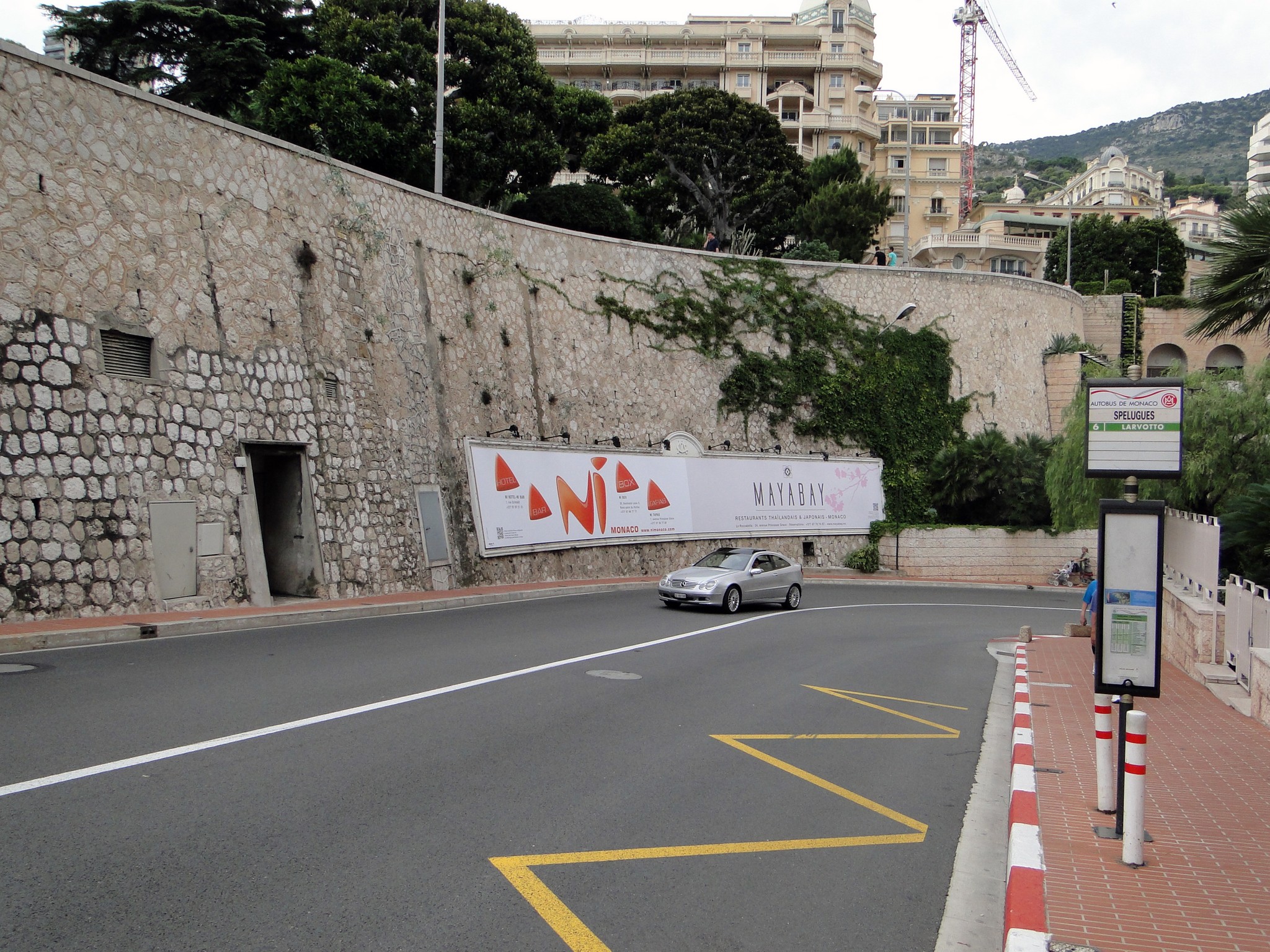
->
[[1077, 583, 1083, 586]]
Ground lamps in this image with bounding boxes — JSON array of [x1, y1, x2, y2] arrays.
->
[[486, 425, 520, 438], [761, 445, 781, 454], [648, 440, 670, 450], [541, 432, 570, 444], [856, 450, 875, 458], [809, 451, 829, 461], [708, 440, 730, 451], [594, 436, 621, 447]]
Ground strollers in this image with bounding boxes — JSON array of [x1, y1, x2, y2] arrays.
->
[[1046, 560, 1078, 587]]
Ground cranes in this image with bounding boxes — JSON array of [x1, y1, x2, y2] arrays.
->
[[952, 0, 1037, 233]]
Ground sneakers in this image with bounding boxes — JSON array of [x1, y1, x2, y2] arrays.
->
[[1111, 695, 1121, 703], [1092, 664, 1095, 675]]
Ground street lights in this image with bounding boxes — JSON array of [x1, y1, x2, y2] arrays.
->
[[854, 86, 910, 267], [1023, 173, 1071, 281]]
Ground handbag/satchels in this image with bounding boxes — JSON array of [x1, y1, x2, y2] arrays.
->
[[1071, 560, 1080, 573]]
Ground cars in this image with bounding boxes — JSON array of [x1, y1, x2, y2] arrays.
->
[[658, 547, 804, 614]]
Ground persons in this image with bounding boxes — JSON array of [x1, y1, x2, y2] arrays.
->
[[1080, 580, 1121, 704], [1074, 546, 1095, 586], [752, 558, 764, 572], [886, 246, 897, 266], [703, 231, 720, 253], [871, 246, 886, 266], [1053, 565, 1070, 575]]
